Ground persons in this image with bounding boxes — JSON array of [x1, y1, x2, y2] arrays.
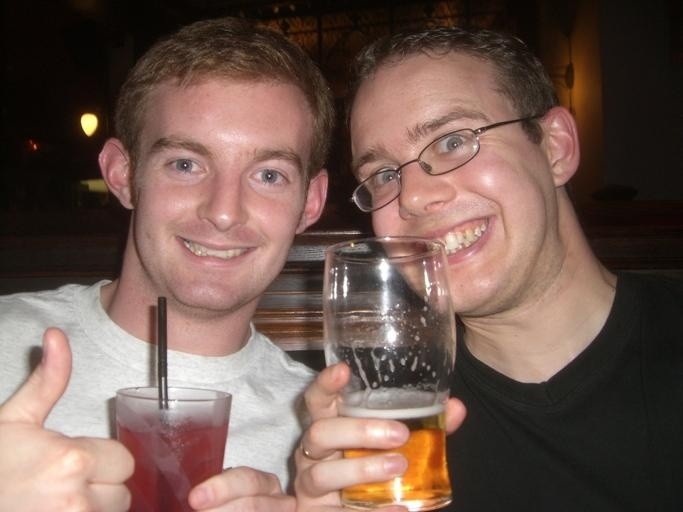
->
[[290, 26, 681, 511], [1, 19, 335, 512]]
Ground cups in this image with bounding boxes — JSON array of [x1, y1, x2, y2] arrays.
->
[[320, 237, 458, 511], [114, 386, 232, 511]]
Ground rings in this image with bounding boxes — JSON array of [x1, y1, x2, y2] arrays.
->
[[300, 435, 326, 461]]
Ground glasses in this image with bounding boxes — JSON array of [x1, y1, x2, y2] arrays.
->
[[348, 115, 542, 214]]
